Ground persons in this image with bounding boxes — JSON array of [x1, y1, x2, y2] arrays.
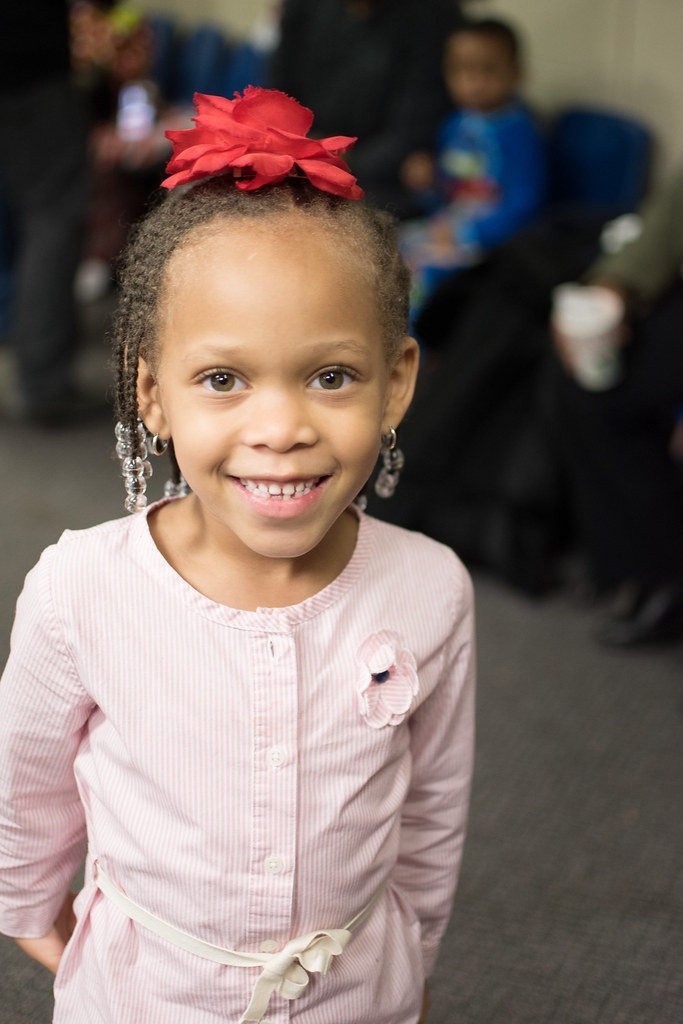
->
[[0, 0, 682, 647], [0, 85, 476, 1024]]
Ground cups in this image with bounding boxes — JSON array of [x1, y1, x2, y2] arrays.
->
[[551, 283, 624, 391]]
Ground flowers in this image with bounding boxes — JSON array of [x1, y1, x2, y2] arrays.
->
[[157, 85, 366, 206]]
